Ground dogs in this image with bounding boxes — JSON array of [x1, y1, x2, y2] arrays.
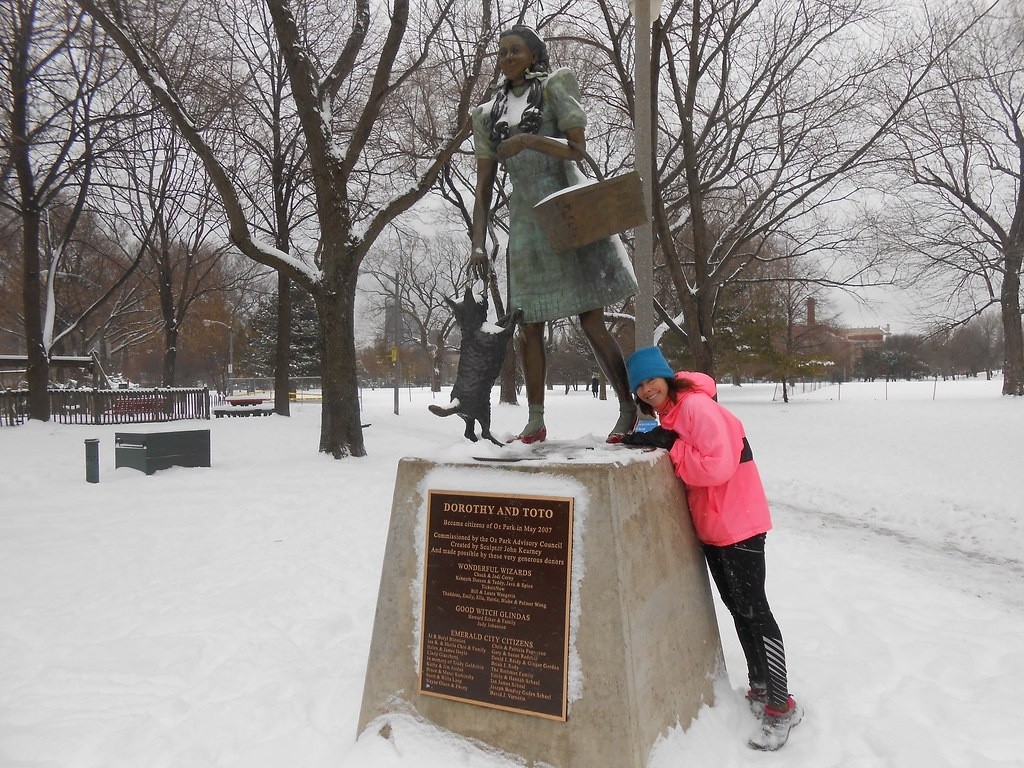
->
[[427, 285, 524, 447]]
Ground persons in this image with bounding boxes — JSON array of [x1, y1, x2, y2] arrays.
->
[[468, 25, 639, 444], [622, 347, 804, 751], [592, 378, 599, 399]]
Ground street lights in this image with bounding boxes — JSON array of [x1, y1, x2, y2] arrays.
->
[[203, 320, 233, 396]]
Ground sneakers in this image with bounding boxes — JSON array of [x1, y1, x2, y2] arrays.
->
[[742, 692, 769, 718], [750, 693, 804, 754]]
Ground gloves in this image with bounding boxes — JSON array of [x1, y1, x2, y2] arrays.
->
[[622, 424, 678, 449]]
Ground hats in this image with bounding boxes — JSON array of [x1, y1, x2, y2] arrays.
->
[[625, 346, 677, 394]]
